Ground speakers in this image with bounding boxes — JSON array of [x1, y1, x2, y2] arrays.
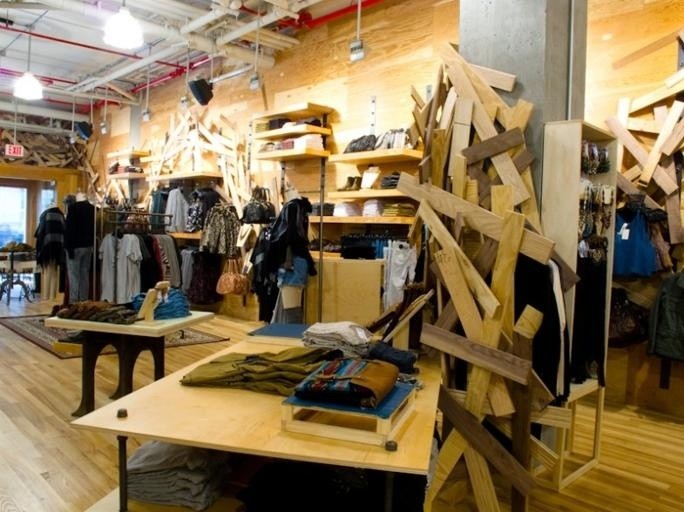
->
[[74, 119, 93, 137], [188, 79, 214, 105]]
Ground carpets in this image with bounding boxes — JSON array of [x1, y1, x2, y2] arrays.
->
[[0, 315, 230, 361]]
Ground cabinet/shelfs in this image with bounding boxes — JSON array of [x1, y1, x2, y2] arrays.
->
[[252, 102, 425, 262], [103, 149, 224, 241], [539, 119, 618, 494]]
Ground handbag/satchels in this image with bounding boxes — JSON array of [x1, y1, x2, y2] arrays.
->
[[216, 259, 250, 296], [240, 188, 276, 223], [375, 130, 413, 149], [294, 358, 399, 408], [344, 136, 375, 153]]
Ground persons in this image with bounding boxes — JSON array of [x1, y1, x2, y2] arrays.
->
[[65, 192, 98, 304], [269, 183, 310, 326]]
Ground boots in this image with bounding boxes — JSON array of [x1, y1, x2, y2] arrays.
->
[[337, 177, 361, 191]]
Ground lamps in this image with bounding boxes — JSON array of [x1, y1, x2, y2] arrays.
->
[[102, 1, 144, 49], [140, 65, 154, 121], [349, 1, 365, 62], [68, 85, 110, 145], [13, 24, 44, 101], [187, 42, 215, 106], [248, 6, 263, 91]]
